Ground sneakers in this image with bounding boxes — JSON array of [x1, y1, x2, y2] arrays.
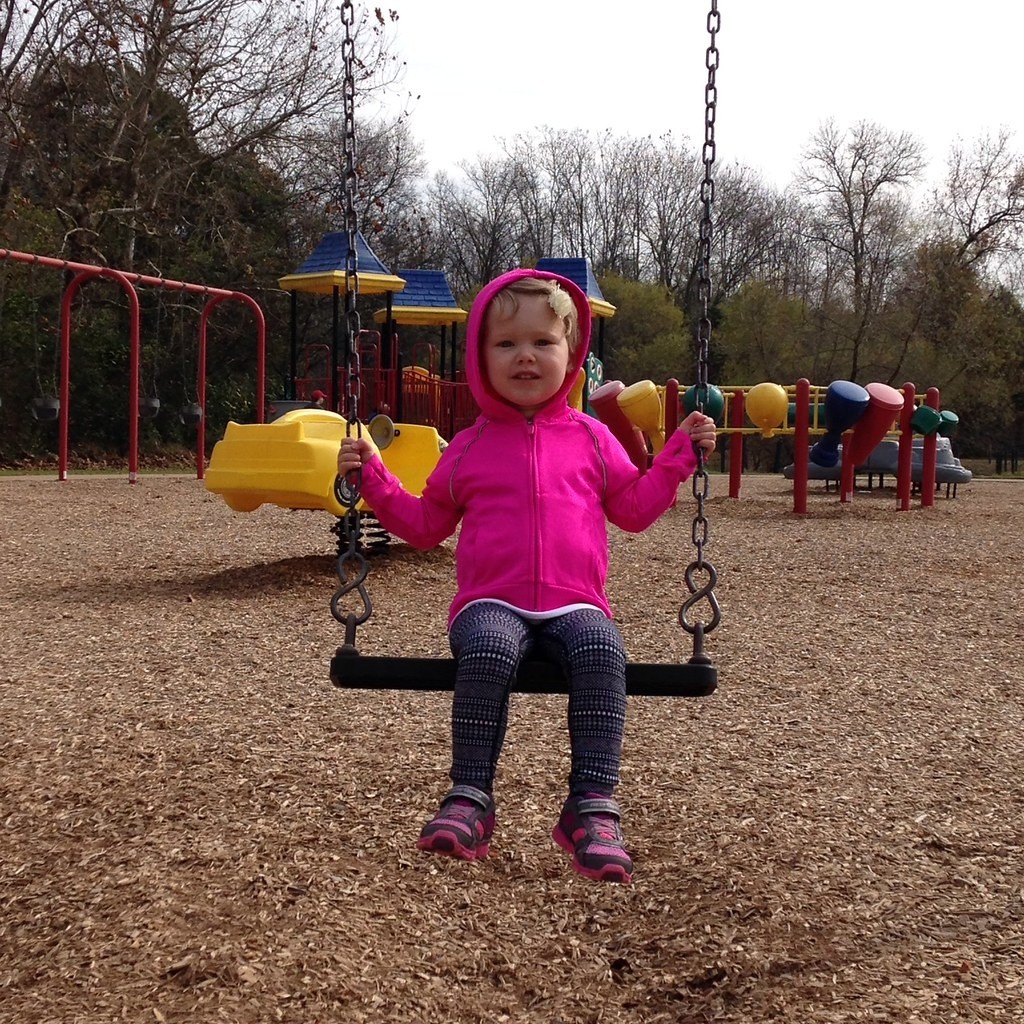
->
[[416, 783, 496, 862], [552, 790, 634, 884]]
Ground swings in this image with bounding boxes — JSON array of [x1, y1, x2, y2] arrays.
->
[[323, 0, 724, 701]]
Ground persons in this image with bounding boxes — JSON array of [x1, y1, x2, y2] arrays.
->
[[338, 268, 717, 881], [304, 390, 327, 409], [369, 401, 390, 424]]
